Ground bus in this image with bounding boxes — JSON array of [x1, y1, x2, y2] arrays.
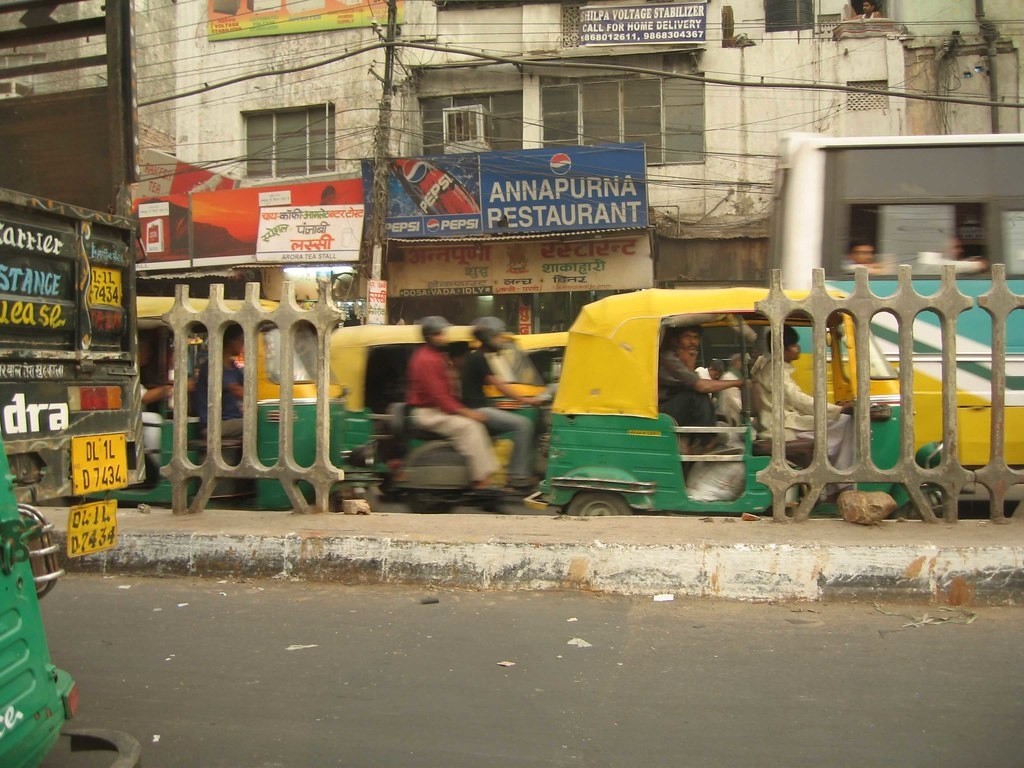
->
[[770, 133, 1024, 519]]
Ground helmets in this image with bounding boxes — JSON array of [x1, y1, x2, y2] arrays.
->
[[472, 316, 507, 339], [422, 315, 454, 333]]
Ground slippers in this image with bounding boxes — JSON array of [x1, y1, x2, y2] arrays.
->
[[470, 484, 514, 495]]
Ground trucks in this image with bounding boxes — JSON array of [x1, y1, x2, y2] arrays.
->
[[0, 0, 146, 601]]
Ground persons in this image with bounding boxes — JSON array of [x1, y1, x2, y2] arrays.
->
[[408, 316, 554, 490], [192, 321, 243, 440], [939, 236, 989, 273], [842, 241, 881, 273], [660, 326, 856, 502], [138, 333, 173, 411]]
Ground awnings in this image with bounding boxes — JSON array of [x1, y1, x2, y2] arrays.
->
[[386, 224, 656, 243], [139, 265, 283, 280]]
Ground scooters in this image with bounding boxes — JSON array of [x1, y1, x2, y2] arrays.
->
[[344, 397, 558, 515]]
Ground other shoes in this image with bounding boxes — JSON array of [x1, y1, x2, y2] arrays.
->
[[511, 477, 540, 487]]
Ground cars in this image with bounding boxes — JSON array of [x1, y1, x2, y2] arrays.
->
[[526, 286, 946, 522], [78, 298, 351, 511], [0, 438, 144, 768], [502, 331, 570, 459], [328, 323, 547, 510]]
